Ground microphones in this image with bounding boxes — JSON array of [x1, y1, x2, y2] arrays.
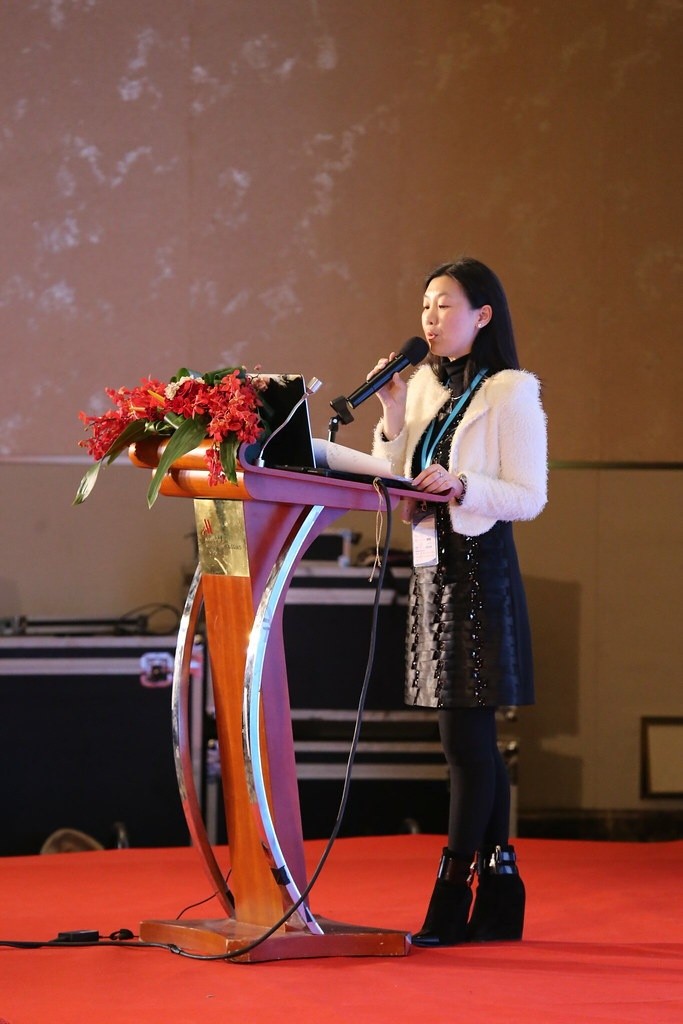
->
[[347, 337, 429, 409]]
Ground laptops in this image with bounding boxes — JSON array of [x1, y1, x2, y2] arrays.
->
[[242, 373, 420, 492]]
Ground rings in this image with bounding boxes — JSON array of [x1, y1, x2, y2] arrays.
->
[[437, 469, 443, 477]]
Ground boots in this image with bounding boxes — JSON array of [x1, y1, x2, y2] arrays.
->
[[412, 846, 474, 947], [464, 843, 525, 941]]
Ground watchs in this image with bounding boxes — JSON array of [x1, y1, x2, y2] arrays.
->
[[453, 475, 468, 505]]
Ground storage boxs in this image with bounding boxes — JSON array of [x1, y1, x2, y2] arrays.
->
[[0, 528, 520, 856]]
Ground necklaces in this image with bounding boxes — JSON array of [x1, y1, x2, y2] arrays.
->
[[445, 395, 463, 414]]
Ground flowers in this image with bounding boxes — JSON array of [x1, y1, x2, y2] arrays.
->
[[72, 365, 269, 510]]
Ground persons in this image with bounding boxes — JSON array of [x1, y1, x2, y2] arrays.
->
[[367, 256, 549, 947]]
